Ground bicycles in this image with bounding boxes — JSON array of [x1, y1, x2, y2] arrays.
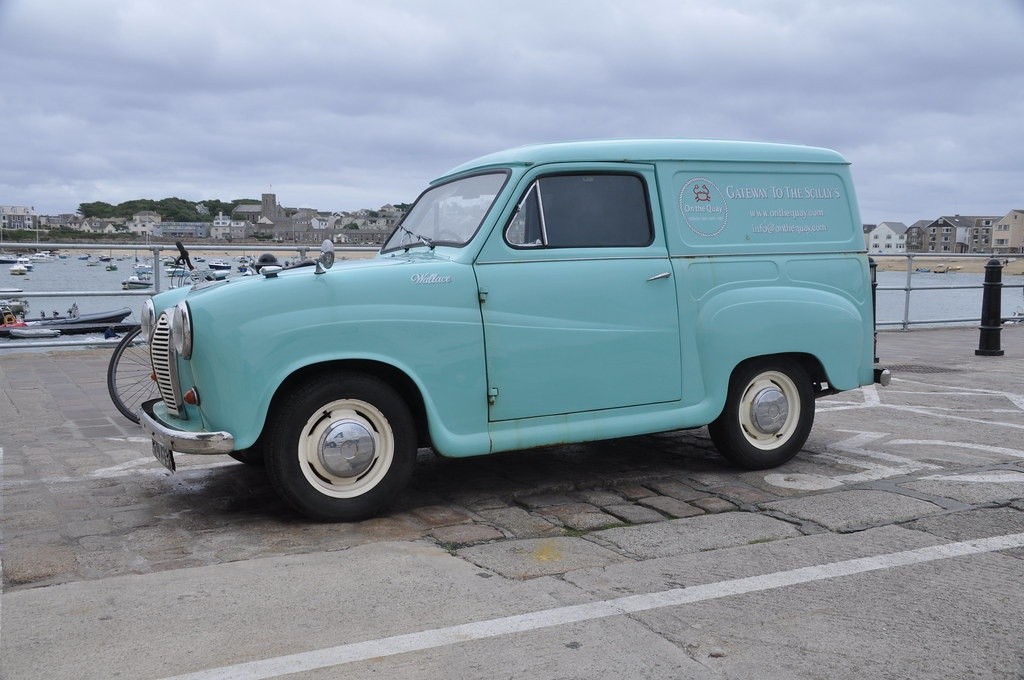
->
[[109, 241, 214, 424]]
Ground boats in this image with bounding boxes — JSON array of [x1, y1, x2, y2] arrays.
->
[[193, 256, 206, 263], [236, 235, 257, 272], [78, 255, 89, 259], [159, 256, 190, 277], [127, 253, 154, 289], [919, 266, 946, 274], [0, 289, 132, 338], [0, 250, 67, 275], [208, 259, 231, 269], [87, 248, 132, 271]]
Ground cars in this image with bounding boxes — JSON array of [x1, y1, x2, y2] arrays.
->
[[140, 138, 890, 524]]
